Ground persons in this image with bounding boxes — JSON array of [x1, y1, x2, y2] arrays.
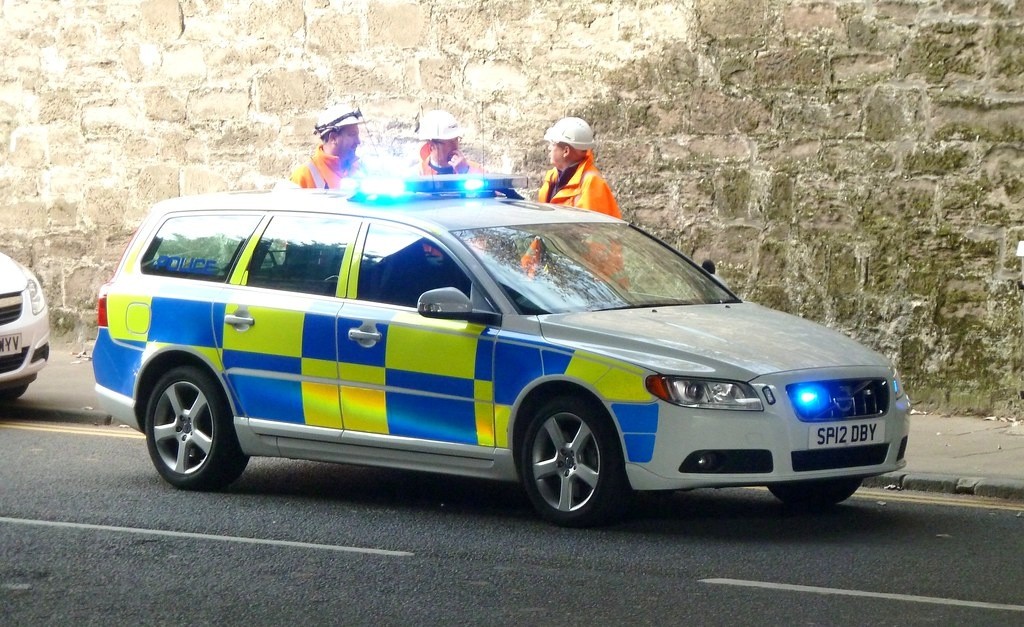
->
[[287, 104, 366, 189], [410, 109, 488, 255], [519, 116, 628, 290]]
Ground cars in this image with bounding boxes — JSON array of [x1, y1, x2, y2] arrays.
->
[[90, 173, 912, 532], [0, 253, 53, 408]]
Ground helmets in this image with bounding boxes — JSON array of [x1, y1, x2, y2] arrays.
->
[[545, 117, 595, 150], [420, 109, 465, 141], [315, 106, 367, 136]]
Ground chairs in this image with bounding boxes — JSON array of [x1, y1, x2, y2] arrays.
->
[[369, 242, 433, 308], [269, 243, 326, 295]]
